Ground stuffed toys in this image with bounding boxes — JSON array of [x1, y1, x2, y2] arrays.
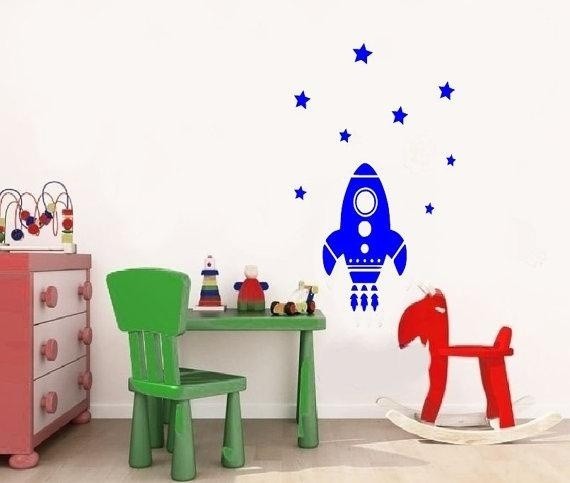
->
[[232, 264, 269, 313]]
